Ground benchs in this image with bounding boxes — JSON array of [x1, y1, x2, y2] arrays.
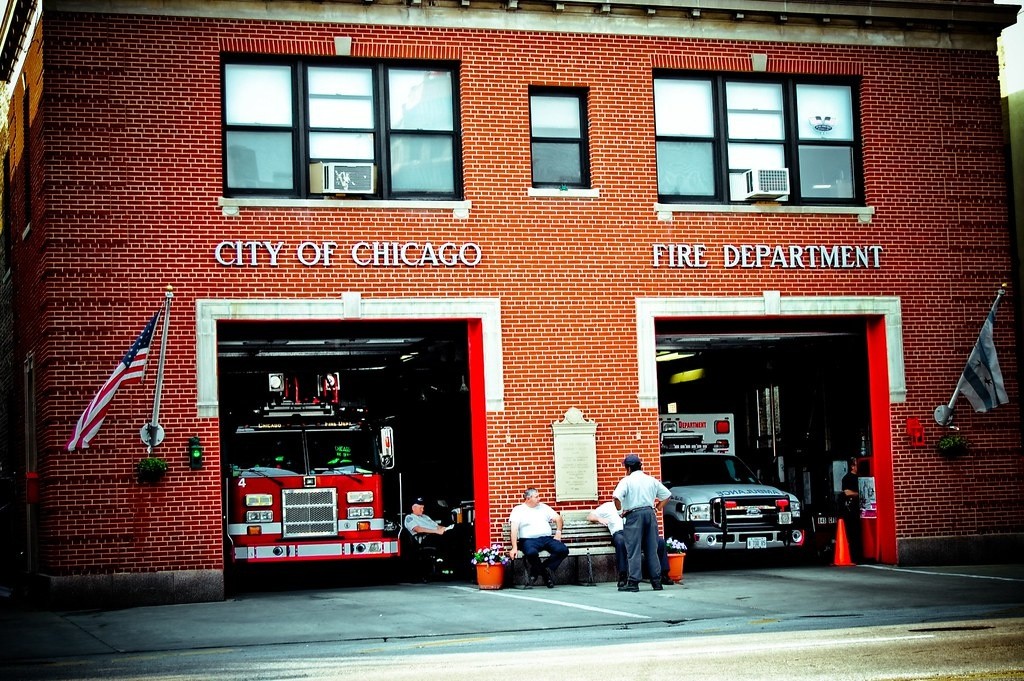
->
[[501, 509, 643, 589]]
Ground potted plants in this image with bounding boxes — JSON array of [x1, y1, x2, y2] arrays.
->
[[937, 434, 968, 459], [138, 459, 167, 483]]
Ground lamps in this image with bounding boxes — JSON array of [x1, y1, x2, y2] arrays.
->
[[656, 351, 697, 361], [189, 437, 202, 469]]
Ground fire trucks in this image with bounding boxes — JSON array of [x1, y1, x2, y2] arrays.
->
[[655, 413, 804, 569], [224, 365, 409, 596]]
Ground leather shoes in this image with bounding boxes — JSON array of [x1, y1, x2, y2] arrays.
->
[[617, 571, 628, 587], [660, 573, 674, 585]]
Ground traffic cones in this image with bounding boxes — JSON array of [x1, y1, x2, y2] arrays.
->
[[828, 518, 857, 567]]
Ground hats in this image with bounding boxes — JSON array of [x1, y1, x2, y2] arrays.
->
[[622, 454, 642, 465], [411, 497, 424, 506]]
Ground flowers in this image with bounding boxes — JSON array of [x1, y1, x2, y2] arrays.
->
[[665, 536, 688, 554], [472, 544, 510, 566]]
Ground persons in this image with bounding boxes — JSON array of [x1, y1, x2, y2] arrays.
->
[[842, 457, 862, 563], [404, 496, 467, 547], [587, 502, 674, 587], [509, 488, 570, 588], [612, 455, 672, 592]]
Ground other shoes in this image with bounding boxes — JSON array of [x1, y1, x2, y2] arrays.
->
[[530, 571, 539, 582], [654, 583, 663, 590], [541, 567, 553, 588], [617, 580, 639, 592]]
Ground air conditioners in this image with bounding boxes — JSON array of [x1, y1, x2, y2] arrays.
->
[[742, 168, 790, 198], [319, 162, 377, 196]]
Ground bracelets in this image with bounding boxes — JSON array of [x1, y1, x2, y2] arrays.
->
[[617, 510, 622, 515], [556, 530, 562, 533]]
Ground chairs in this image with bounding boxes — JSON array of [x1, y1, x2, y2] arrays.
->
[[396, 513, 434, 581]]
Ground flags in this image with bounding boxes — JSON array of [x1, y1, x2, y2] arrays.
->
[[958, 307, 1010, 413], [63, 308, 162, 451]]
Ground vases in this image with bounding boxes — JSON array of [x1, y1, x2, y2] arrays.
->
[[664, 552, 686, 582], [476, 563, 503, 589]]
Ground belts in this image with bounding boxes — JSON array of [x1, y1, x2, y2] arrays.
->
[[626, 507, 653, 515]]
